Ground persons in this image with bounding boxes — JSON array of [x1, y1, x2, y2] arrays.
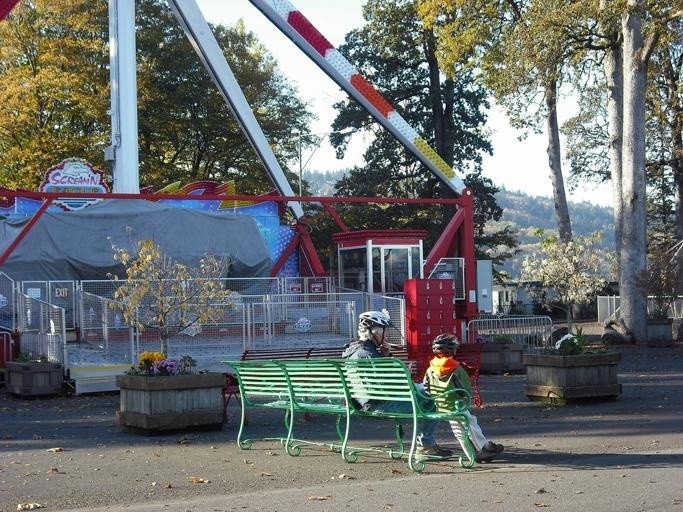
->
[[423, 334, 504, 463], [341, 311, 453, 461]]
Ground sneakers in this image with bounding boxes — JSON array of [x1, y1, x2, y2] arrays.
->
[[475, 441, 503, 463], [415, 444, 453, 462]]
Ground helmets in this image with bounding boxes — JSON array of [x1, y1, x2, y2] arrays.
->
[[432, 333, 461, 355], [358, 311, 395, 330]]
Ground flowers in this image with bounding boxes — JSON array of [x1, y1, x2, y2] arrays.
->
[[137, 350, 181, 376]]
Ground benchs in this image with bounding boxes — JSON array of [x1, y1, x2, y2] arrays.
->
[[220, 341, 488, 473]]
